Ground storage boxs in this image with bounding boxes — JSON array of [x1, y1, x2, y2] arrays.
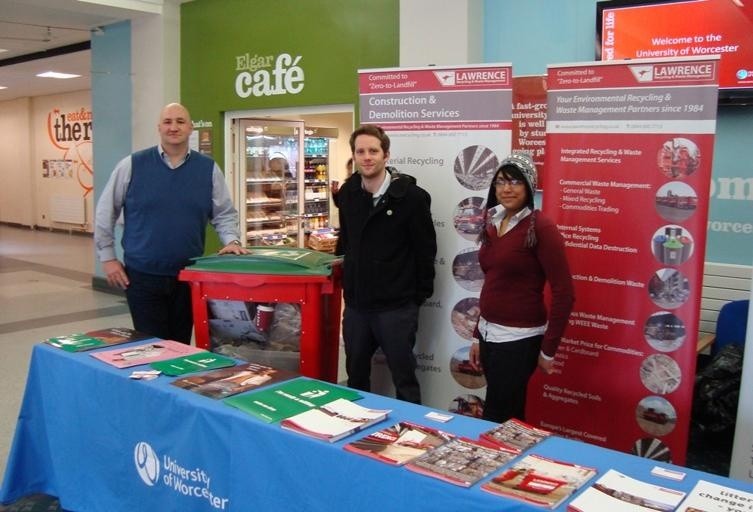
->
[[178, 259, 345, 384]]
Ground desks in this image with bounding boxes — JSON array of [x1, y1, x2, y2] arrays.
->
[[0, 325, 753, 511]]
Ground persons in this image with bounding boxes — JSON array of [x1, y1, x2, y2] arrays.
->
[[92, 104, 253, 346], [334, 124, 436, 406], [470, 153, 575, 425], [332, 158, 353, 255]]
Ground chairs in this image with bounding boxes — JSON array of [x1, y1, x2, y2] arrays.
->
[[688, 299, 753, 477]]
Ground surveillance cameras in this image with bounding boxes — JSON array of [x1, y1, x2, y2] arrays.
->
[[42, 27, 52, 42], [89, 27, 105, 35]]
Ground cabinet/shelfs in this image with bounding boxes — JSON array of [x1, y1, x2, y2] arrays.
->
[[245, 131, 330, 248]]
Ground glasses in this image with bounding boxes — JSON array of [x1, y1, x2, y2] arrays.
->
[[493, 180, 522, 188]]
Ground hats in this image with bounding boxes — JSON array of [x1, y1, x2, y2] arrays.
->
[[476, 154, 537, 247]]
